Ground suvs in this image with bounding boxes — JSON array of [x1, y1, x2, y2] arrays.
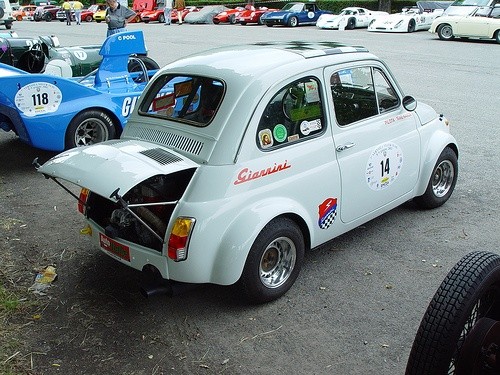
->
[[0, 0, 16, 29]]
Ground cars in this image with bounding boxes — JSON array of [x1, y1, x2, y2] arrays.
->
[[12, 1, 248, 25], [30, 38, 460, 306], [367, 0, 500, 44]]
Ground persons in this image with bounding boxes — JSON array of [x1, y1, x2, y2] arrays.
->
[[175, 0, 185, 25], [63, 0, 71, 25], [73, 0, 83, 25], [105, 0, 137, 37], [163, 0, 174, 25]]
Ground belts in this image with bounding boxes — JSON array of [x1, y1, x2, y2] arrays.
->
[[107, 26, 125, 30]]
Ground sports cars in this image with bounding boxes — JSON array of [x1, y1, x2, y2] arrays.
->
[[259, 2, 334, 28], [316, 7, 390, 30], [0, 27, 221, 154], [234, 6, 281, 25]]
